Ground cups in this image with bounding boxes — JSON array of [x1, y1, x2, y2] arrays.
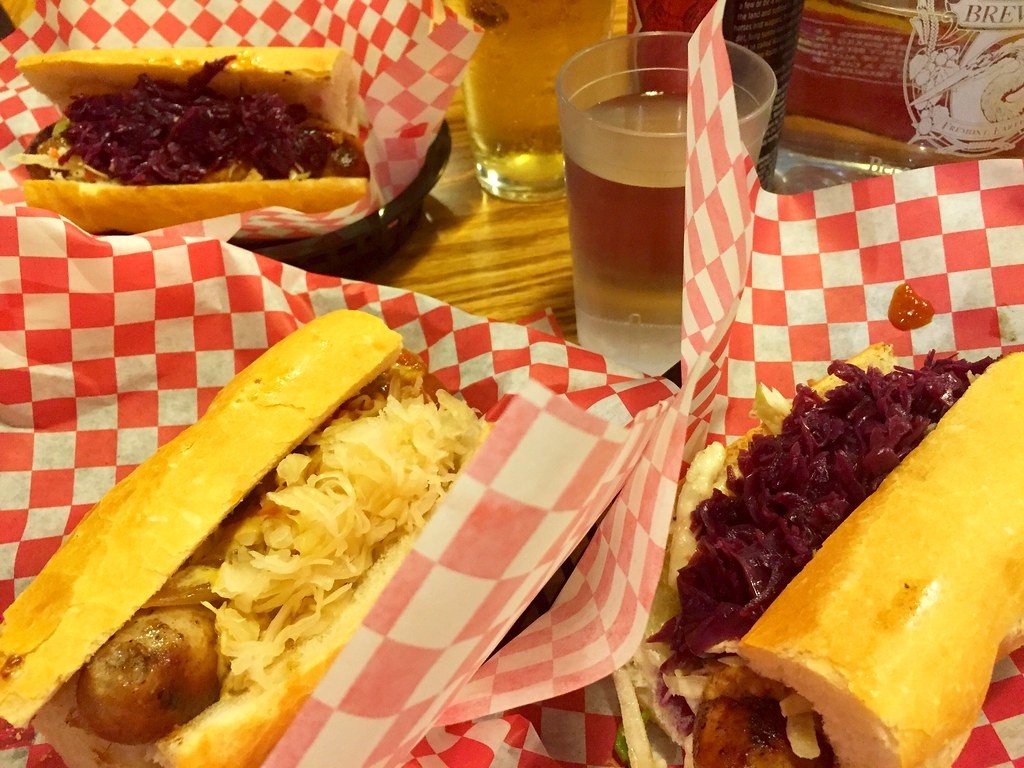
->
[[567, 47, 780, 379], [460, 0, 631, 203]]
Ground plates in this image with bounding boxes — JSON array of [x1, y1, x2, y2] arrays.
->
[[96, 112, 453, 296]]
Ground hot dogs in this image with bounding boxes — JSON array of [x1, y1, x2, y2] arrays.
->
[[12, 44, 373, 237], [611, 341, 1024, 768], [2, 307, 501, 768]]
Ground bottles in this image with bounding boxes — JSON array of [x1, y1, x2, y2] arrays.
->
[[621, 0, 805, 211]]
